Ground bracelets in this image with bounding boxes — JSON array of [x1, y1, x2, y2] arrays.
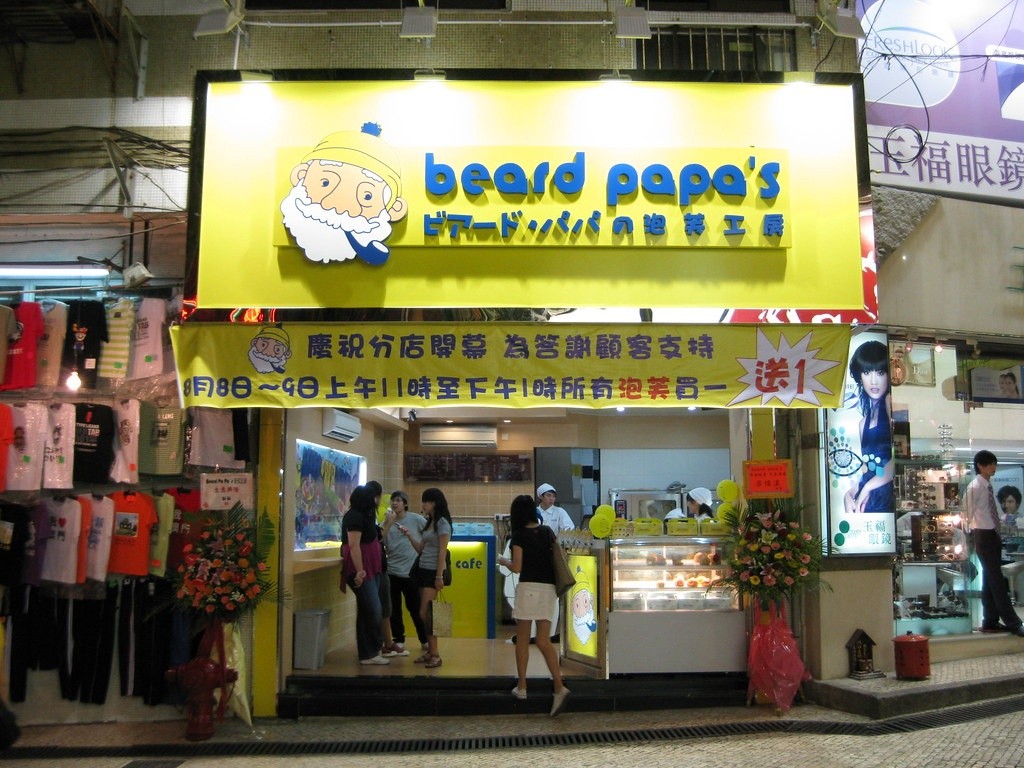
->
[[435, 576, 443, 579], [357, 570, 366, 578]]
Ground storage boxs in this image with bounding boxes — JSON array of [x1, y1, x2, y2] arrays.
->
[[608, 518, 663, 539], [667, 518, 698, 535], [701, 518, 732, 535]]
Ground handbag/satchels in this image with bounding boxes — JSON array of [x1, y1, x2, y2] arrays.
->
[[547, 526, 576, 597], [426, 590, 452, 636]]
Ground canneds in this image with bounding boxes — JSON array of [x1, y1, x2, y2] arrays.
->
[[646, 553, 655, 565]]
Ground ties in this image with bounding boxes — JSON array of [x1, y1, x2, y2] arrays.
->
[[988, 482, 1001, 531]]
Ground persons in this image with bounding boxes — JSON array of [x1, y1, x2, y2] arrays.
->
[[999, 373, 1020, 399], [686, 487, 715, 534], [843, 340, 895, 512], [536, 483, 574, 560], [961, 450, 1024, 637], [382, 488, 453, 669], [996, 486, 1024, 535], [340, 480, 410, 664], [664, 508, 686, 534], [498, 483, 575, 716]]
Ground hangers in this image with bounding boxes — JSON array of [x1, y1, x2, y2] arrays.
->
[[6, 287, 143, 309], [12, 392, 172, 409], [24, 479, 192, 503]]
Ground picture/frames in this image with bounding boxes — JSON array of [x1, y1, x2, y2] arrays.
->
[[888, 345, 936, 387]]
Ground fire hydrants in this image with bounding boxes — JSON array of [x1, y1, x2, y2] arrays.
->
[[163, 657, 239, 742]]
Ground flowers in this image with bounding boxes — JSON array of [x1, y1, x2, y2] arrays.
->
[[146, 501, 293, 637], [705, 498, 834, 611]]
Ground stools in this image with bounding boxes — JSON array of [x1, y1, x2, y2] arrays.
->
[[936, 567, 967, 592], [1000, 561, 1024, 607]]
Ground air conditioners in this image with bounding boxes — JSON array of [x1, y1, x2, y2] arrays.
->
[[419, 424, 498, 449], [322, 407, 361, 443]]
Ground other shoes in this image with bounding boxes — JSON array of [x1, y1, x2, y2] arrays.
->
[[425, 655, 442, 668], [511, 687, 527, 699], [414, 652, 432, 663], [391, 643, 409, 655], [382, 648, 397, 656], [550, 687, 571, 717], [361, 656, 390, 665], [422, 645, 429, 651]]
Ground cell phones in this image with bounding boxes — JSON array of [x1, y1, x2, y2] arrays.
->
[[395, 522, 407, 532]]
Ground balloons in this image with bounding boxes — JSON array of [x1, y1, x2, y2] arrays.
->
[[588, 505, 615, 538], [375, 507, 388, 524], [716, 480, 738, 524], [378, 495, 393, 508]]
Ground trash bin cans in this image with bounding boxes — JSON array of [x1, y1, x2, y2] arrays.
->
[[293, 608, 331, 670]]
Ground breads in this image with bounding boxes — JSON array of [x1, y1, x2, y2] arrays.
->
[[657, 579, 724, 588], [694, 552, 719, 565]]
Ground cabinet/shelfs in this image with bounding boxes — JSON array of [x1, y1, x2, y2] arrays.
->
[[892, 456, 972, 637], [608, 535, 748, 679]]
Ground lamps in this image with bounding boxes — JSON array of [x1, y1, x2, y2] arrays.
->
[[191, 6, 246, 38], [0, 265, 112, 276], [934, 335, 948, 353], [966, 338, 980, 355], [815, 4, 867, 40], [903, 333, 918, 352], [399, 7, 438, 37], [123, 262, 156, 289], [611, 7, 652, 39]]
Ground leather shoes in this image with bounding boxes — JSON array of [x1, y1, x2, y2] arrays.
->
[[981, 622, 1007, 632], [1011, 623, 1024, 636]]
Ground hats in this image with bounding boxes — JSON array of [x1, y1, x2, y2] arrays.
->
[[665, 508, 686, 519], [537, 483, 556, 499], [392, 491, 408, 511], [688, 487, 713, 507]]
